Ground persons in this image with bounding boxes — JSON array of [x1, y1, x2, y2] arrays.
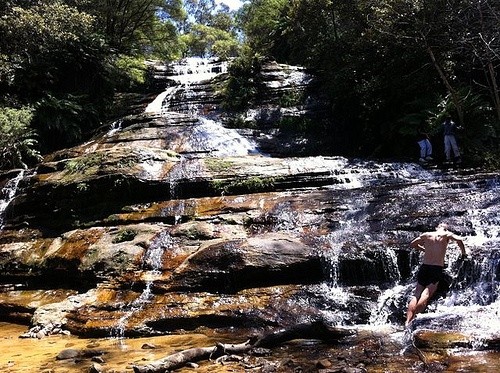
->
[[405, 223, 467, 328], [440, 114, 461, 161], [415, 120, 433, 162]]
